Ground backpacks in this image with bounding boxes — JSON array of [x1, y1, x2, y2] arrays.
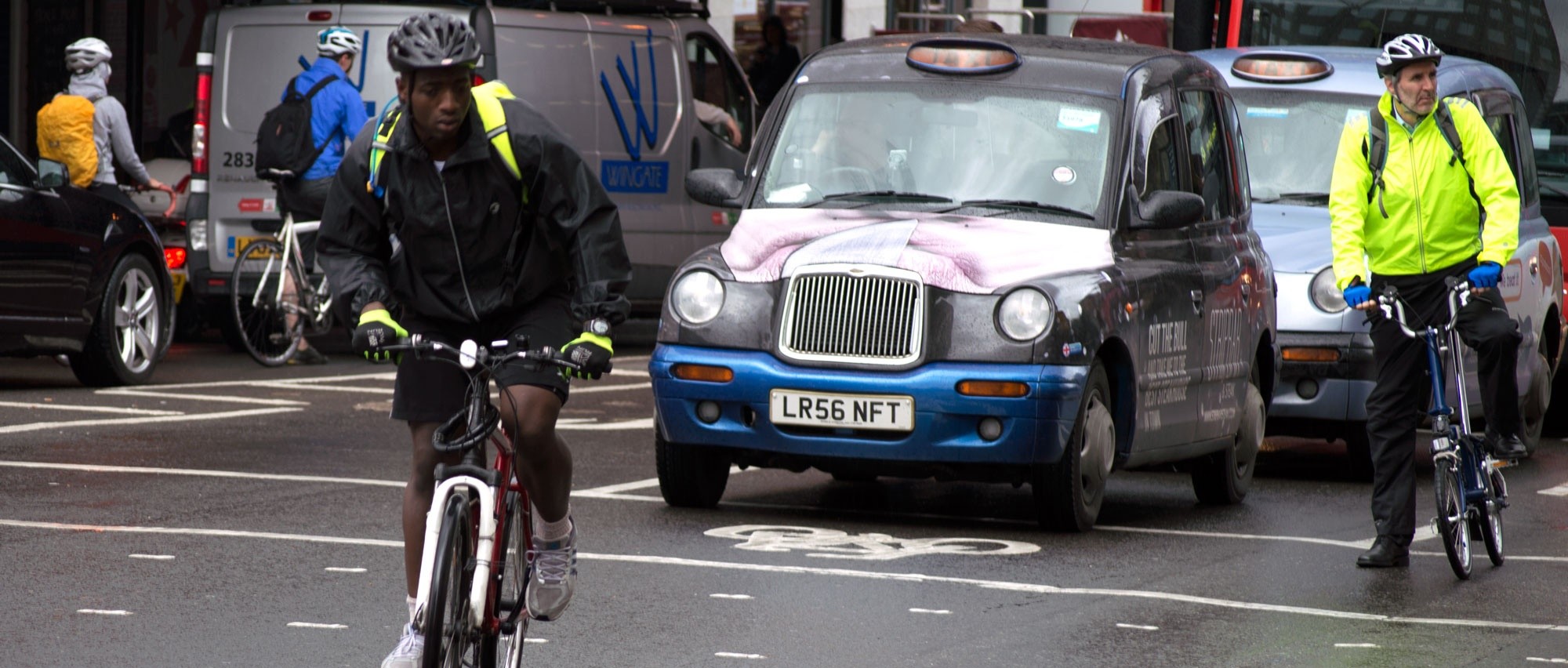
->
[[368, 81, 529, 203], [254, 73, 345, 182], [36, 93, 107, 189]]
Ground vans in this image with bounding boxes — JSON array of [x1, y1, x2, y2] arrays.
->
[[186, 3, 763, 355]]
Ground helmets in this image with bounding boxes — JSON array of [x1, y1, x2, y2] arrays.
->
[[317, 24, 363, 58], [66, 37, 113, 73], [386, 9, 483, 73], [1375, 33, 1441, 78]]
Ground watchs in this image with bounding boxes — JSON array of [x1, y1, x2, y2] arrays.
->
[[582, 318, 613, 336]]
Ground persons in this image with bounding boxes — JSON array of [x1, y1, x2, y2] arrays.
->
[[315, 11, 632, 668], [281, 24, 368, 363], [953, 19, 1003, 33], [1327, 33, 1525, 568], [36, 36, 162, 212], [748, 14, 801, 119], [692, 96, 743, 147]]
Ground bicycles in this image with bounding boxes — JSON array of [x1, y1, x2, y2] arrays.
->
[[382, 339, 613, 667], [229, 198, 335, 367], [118, 184, 177, 218], [1355, 274, 1520, 579]]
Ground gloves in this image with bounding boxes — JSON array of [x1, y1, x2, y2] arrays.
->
[[559, 332, 614, 381], [352, 310, 409, 364]]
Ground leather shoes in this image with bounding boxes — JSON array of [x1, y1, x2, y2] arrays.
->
[[1357, 538, 1410, 569], [1483, 426, 1528, 460]]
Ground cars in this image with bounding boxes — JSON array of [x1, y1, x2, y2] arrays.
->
[[646, 30, 1568, 535], [0, 137, 194, 388]]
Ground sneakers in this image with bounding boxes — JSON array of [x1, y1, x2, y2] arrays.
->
[[289, 345, 328, 364], [525, 516, 576, 621], [379, 621, 426, 668]]
